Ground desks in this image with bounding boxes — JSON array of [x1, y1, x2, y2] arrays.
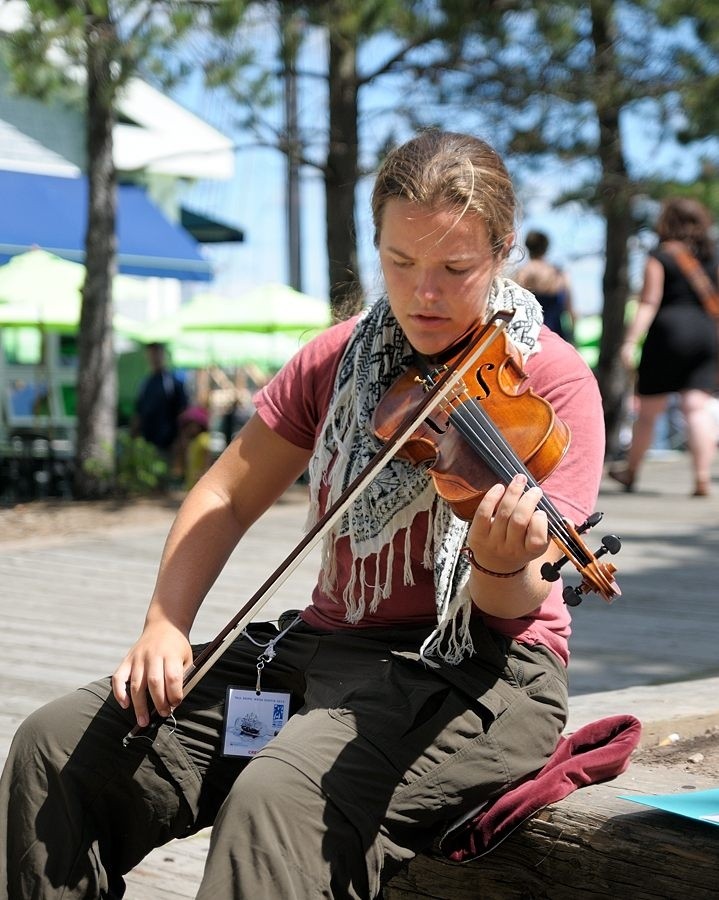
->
[[11, 417, 76, 503]]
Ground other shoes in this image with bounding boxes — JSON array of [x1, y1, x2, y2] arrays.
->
[[693, 481, 710, 496], [607, 470, 637, 491]]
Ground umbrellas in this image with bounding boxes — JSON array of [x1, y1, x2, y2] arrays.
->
[[0, 248, 339, 394]]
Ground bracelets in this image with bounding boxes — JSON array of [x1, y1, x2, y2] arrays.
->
[[459, 546, 528, 578]]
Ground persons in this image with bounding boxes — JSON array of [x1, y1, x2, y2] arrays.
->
[[126, 343, 193, 472], [602, 201, 719, 498], [0, 132, 605, 900], [511, 230, 578, 343]]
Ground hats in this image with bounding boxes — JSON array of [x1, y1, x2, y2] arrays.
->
[[179, 406, 210, 431]]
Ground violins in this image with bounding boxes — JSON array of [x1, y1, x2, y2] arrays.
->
[[371, 323, 624, 609]]
